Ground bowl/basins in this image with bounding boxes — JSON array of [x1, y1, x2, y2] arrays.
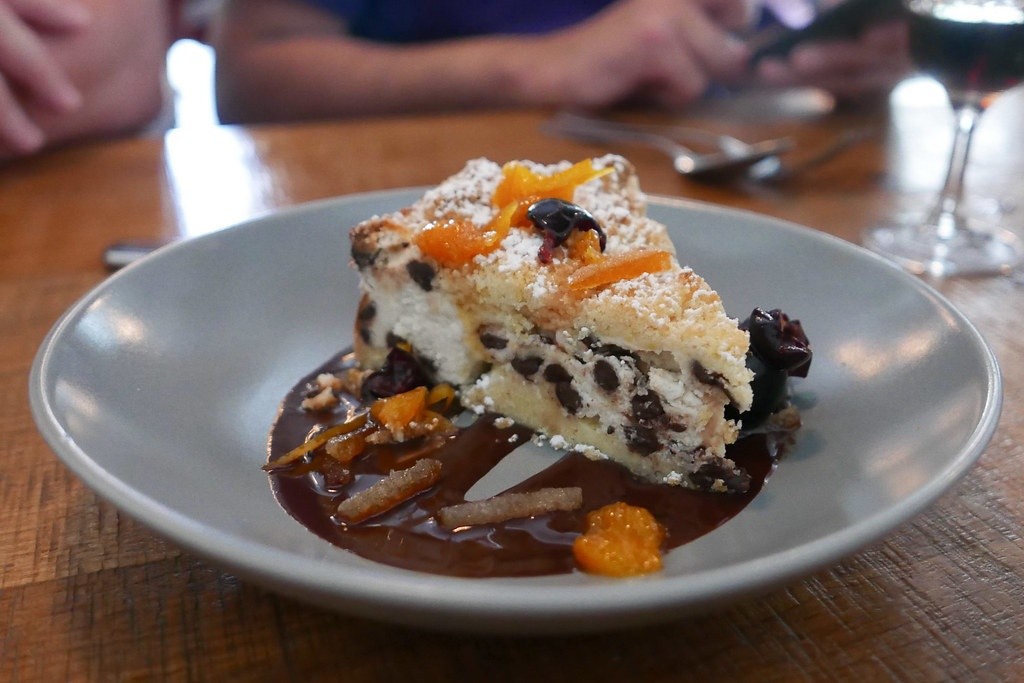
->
[[24, 184, 1005, 636]]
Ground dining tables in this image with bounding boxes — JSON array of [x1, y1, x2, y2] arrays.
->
[[0, 111, 1024, 683]]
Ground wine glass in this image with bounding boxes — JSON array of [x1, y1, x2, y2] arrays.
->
[[860, 0, 1024, 276]]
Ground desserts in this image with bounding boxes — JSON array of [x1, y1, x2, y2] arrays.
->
[[347, 153, 756, 495]]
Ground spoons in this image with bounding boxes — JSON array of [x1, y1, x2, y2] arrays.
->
[[548, 121, 796, 179]]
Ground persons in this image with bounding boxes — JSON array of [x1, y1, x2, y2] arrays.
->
[[213, 1, 916, 125], [0, 0, 170, 172]]
